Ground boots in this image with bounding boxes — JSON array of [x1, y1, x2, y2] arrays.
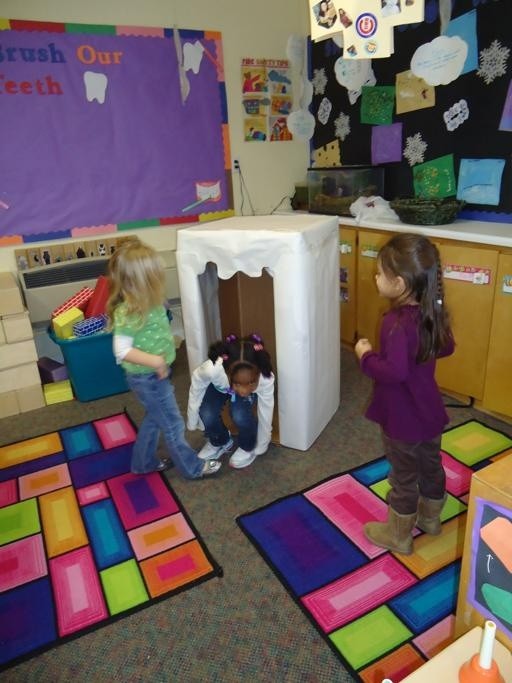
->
[[364, 503, 418, 555], [415, 488, 448, 536]]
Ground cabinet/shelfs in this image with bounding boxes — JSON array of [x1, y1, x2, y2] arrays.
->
[[356, 230, 500, 421], [473, 243, 512, 430], [177, 215, 341, 452], [452, 454, 512, 655], [339, 224, 356, 354]]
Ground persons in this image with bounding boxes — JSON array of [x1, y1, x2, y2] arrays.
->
[[317, 0, 334, 27], [111, 239, 222, 480], [354, 230, 457, 555], [186, 331, 276, 470]]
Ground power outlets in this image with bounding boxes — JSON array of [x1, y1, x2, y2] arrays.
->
[[232, 159, 240, 175]]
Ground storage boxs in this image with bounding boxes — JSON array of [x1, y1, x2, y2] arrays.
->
[[47, 308, 174, 402]]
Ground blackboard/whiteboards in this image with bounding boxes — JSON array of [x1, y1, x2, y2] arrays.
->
[[2, 20, 236, 244], [308, 0, 511, 219]]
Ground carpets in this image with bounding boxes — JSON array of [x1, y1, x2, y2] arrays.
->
[[0, 406, 223, 676], [236, 420, 512, 683]]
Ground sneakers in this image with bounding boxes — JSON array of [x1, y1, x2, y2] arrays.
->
[[200, 460, 222, 474], [198, 436, 233, 460], [156, 457, 174, 471], [229, 446, 257, 468]]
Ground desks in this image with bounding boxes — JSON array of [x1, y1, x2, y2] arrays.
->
[[398, 626, 512, 683]]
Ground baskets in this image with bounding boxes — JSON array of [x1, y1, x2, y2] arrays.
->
[[389, 196, 467, 225]]
